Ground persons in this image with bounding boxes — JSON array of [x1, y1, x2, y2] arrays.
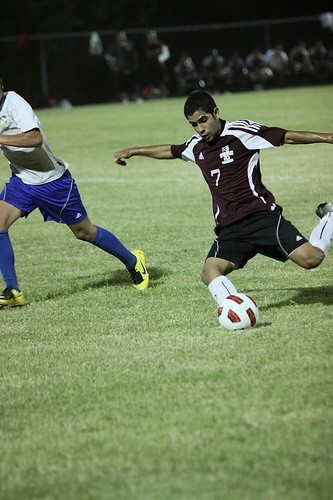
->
[[47, 28, 333, 108], [0, 79, 150, 307], [114, 92, 333, 311]]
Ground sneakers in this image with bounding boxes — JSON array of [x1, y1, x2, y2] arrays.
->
[[315, 202, 333, 219], [0, 288, 25, 306], [126, 249, 149, 290]]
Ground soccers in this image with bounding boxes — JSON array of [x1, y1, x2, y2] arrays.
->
[[215, 292, 261, 331]]
